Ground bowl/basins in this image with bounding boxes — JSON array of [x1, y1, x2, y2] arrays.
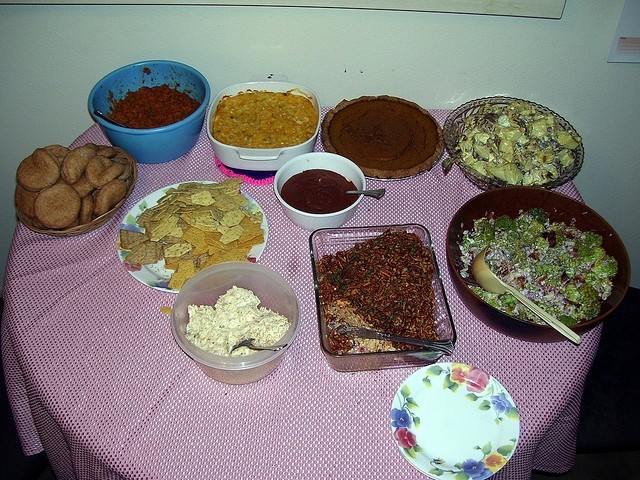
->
[[88, 60, 212, 164], [273, 151, 367, 232], [169, 260, 302, 386], [206, 81, 322, 172], [444, 185, 633, 344]]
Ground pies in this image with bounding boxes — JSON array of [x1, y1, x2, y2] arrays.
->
[[15, 143, 132, 231], [213, 90, 318, 150]]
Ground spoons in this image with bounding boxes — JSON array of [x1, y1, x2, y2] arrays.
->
[[470, 247, 582, 345], [330, 325, 454, 356], [229, 338, 289, 354]]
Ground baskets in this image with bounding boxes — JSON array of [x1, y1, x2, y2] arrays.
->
[[16, 145, 137, 237]]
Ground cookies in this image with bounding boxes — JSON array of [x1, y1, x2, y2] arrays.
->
[[119, 178, 266, 293]]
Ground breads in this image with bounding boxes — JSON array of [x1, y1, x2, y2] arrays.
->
[[323, 95, 445, 178]]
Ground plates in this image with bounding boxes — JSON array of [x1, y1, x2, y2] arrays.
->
[[365, 148, 445, 182], [441, 96, 585, 193], [116, 180, 269, 295], [390, 362, 521, 480]]
